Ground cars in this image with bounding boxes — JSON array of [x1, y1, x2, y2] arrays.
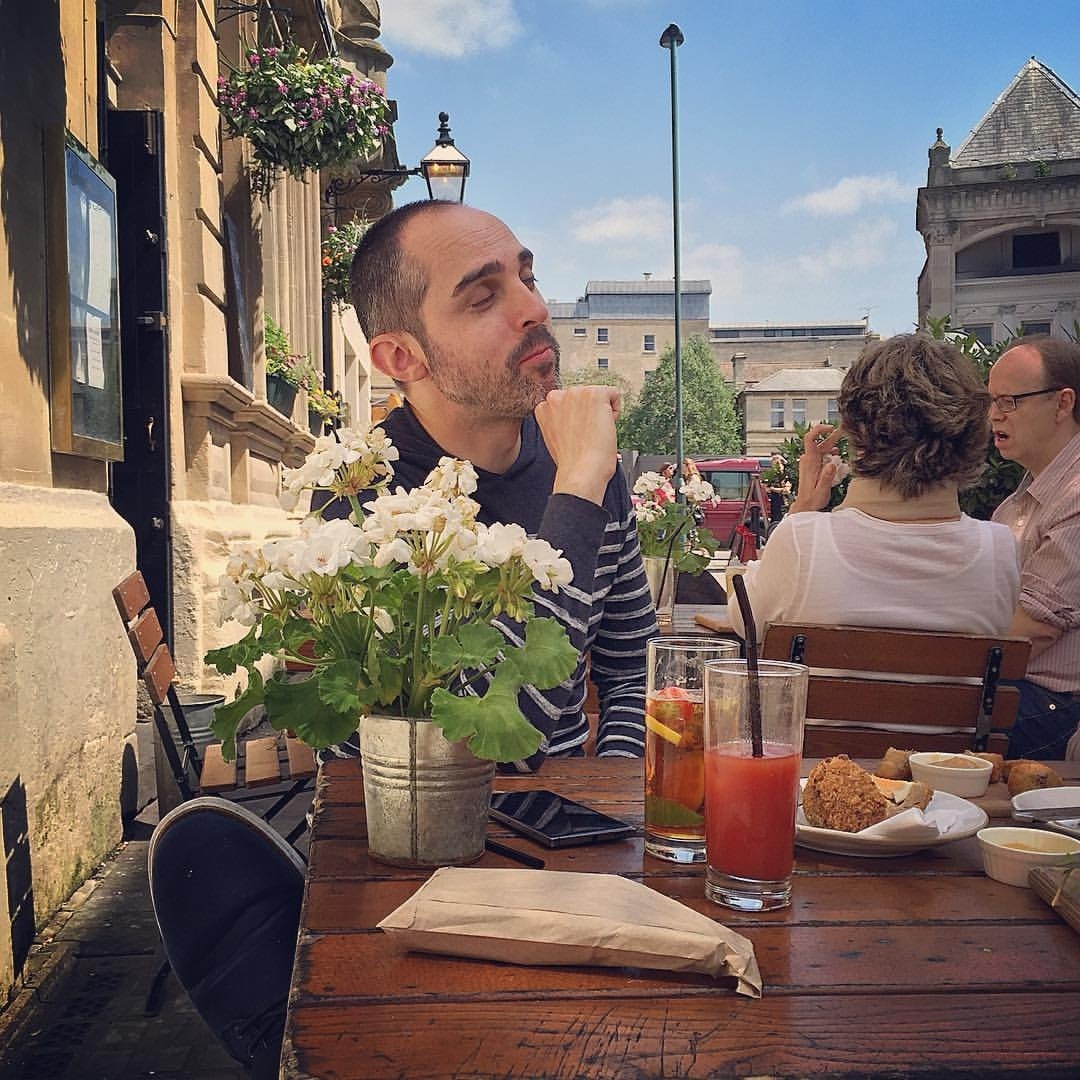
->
[[694, 455, 770, 564]]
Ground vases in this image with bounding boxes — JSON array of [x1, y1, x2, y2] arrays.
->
[[640, 555, 674, 626], [357, 712, 498, 865]]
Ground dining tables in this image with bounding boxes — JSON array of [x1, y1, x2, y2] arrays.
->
[[278, 752, 1080, 1080]]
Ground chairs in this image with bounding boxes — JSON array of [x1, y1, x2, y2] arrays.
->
[[761, 622, 1033, 756], [112, 570, 322, 842]]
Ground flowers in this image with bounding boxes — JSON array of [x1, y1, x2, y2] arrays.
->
[[633, 472, 719, 577], [220, 43, 392, 179], [195, 429, 581, 760], [321, 214, 372, 311]]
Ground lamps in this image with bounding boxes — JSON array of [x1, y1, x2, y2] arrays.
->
[[323, 113, 470, 227]]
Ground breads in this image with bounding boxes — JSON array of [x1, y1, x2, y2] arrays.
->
[[802, 754, 933, 834]]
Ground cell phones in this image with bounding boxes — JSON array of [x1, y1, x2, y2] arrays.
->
[[488, 788, 637, 848]]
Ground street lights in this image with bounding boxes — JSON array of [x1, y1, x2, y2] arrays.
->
[[658, 24, 690, 533]]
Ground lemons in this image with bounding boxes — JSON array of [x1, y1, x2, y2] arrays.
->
[[646, 791, 702, 827]]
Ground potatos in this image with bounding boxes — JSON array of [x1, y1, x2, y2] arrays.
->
[[974, 752, 1064, 795], [877, 746, 914, 779]]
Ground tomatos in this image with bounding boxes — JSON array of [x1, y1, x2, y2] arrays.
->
[[649, 686, 691, 731]]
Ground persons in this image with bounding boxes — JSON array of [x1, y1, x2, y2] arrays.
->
[[296, 198, 661, 779], [679, 456, 708, 517], [730, 329, 1022, 759], [764, 454, 791, 513], [652, 461, 677, 511], [956, 334, 1079, 760]]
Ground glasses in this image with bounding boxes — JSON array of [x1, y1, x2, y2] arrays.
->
[[992, 387, 1063, 413]]
